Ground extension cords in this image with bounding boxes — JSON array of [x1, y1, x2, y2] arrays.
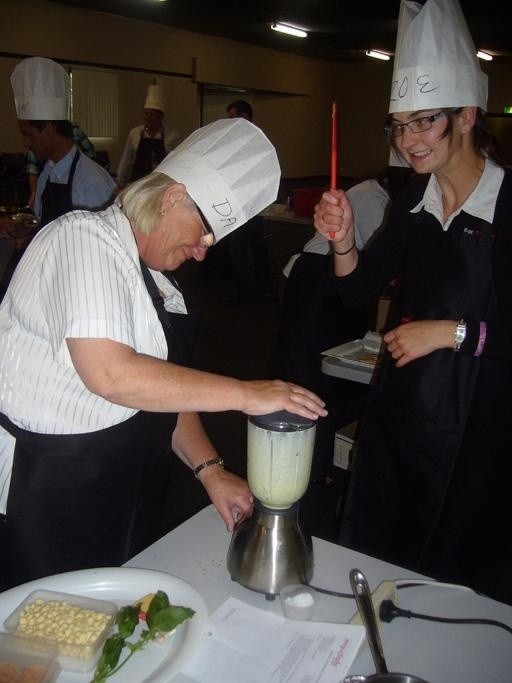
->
[[349, 579, 399, 632]]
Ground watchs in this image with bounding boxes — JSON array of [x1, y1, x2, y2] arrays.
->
[[453, 316, 466, 353]]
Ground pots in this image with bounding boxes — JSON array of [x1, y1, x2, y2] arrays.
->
[[349, 568, 429, 683]]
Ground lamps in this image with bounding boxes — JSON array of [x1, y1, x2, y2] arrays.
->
[[366, 49, 390, 61], [476, 50, 493, 61], [270, 20, 308, 38]]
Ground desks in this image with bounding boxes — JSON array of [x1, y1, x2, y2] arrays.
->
[[256, 203, 313, 270], [120, 498, 511, 683]]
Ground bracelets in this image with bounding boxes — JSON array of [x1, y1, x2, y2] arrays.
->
[[336, 236, 356, 255], [194, 456, 224, 479]]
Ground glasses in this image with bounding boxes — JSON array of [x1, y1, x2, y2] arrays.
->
[[391, 109, 450, 136]]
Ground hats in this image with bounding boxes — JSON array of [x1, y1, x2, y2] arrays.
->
[[143, 87, 165, 110], [389, 0, 488, 114], [10, 57, 69, 121], [153, 119, 282, 245]]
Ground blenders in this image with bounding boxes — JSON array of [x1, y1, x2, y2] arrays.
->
[[226, 409, 318, 601]]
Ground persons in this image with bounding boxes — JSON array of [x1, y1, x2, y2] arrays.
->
[[10, 57, 122, 252], [219, 100, 267, 304], [0, 117, 328, 594], [116, 76, 183, 190], [314, 0, 512, 606], [273, 143, 413, 391], [15, 125, 96, 208]]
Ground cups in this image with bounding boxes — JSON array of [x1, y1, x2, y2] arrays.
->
[[280, 584, 320, 620]]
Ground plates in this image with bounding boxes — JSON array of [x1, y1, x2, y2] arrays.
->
[[0, 567, 208, 683]]
